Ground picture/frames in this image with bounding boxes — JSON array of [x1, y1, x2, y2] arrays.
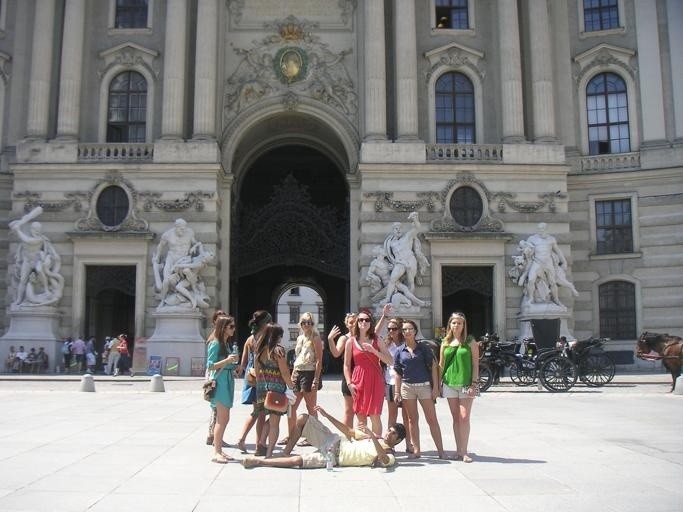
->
[[147, 355, 161, 376], [165, 357, 179, 376], [191, 357, 206, 377]]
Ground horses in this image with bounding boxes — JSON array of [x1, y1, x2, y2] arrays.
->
[[636, 331, 683, 394]]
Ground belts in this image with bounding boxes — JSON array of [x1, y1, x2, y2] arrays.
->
[[406, 382, 430, 386]]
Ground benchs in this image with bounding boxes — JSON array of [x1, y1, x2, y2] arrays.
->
[[5, 357, 45, 373]]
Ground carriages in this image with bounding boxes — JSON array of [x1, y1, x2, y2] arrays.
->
[[415, 332, 618, 396]]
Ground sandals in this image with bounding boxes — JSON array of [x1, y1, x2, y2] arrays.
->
[[276, 437, 288, 445], [405, 444, 473, 463], [296, 439, 312, 446]]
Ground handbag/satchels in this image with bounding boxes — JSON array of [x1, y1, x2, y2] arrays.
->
[[203, 379, 216, 402], [247, 368, 256, 387], [264, 391, 289, 412]]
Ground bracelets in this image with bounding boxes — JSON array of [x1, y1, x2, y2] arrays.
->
[[347, 382, 352, 386]]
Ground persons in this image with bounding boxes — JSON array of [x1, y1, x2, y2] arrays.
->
[[9, 221, 50, 306], [241, 406, 407, 468], [393, 319, 449, 460], [560, 336, 572, 361], [343, 310, 392, 438], [525, 223, 566, 306], [374, 304, 415, 452], [152, 219, 214, 311], [204, 310, 240, 464], [237, 310, 273, 451], [368, 246, 432, 307], [438, 312, 479, 462], [328, 312, 357, 429], [254, 323, 293, 457], [5, 334, 136, 377], [278, 312, 323, 446], [380, 211, 421, 304]]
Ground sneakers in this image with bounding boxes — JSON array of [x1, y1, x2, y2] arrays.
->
[[205, 436, 273, 468], [85, 368, 136, 377]]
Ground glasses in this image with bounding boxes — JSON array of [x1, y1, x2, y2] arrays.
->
[[452, 312, 464, 318], [302, 322, 311, 325], [227, 325, 235, 329], [401, 328, 415, 333], [346, 312, 358, 319], [387, 328, 397, 331], [359, 318, 370, 322]]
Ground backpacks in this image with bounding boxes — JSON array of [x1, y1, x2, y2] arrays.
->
[[397, 338, 440, 385]]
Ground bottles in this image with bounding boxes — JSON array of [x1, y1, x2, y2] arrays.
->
[[231, 341, 238, 364]]
